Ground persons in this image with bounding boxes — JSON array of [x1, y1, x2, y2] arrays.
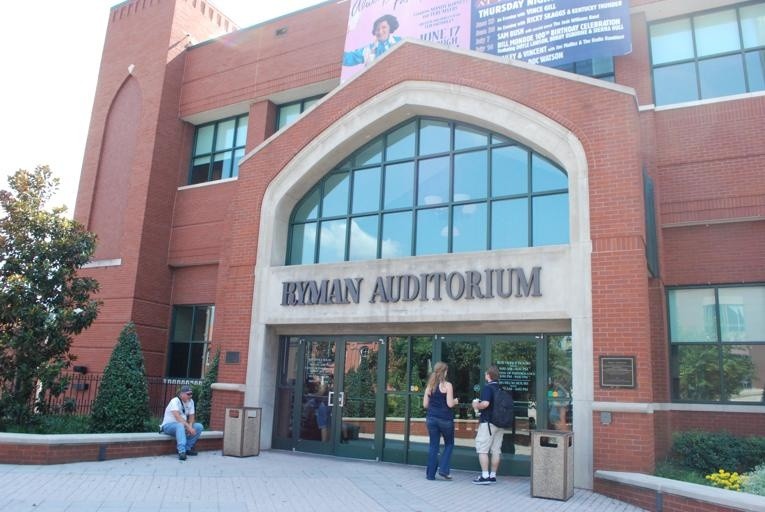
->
[[340, 14, 406, 71], [472, 366, 504, 484], [422, 360, 460, 480], [285, 376, 349, 445], [158, 385, 204, 461]]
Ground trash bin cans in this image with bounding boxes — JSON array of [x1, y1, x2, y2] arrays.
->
[[530, 430, 575, 501], [222, 406, 262, 458]]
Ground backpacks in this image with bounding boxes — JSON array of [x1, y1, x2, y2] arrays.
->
[[486, 384, 513, 428]]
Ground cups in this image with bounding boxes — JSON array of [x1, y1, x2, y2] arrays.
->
[[472, 400, 480, 413]]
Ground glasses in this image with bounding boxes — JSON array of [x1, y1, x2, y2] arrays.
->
[[186, 393, 193, 395]]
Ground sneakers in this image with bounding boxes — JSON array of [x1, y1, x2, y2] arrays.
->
[[472, 477, 496, 484], [186, 449, 197, 455], [439, 472, 452, 479], [179, 452, 186, 460]]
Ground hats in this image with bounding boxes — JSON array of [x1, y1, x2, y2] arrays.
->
[[181, 386, 192, 392]]
